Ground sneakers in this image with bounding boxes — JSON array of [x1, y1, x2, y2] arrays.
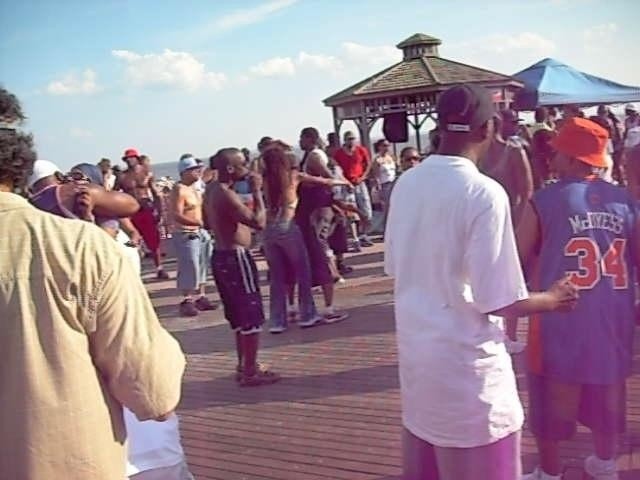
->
[[179, 297, 197, 315], [505, 333, 526, 354], [584, 452, 619, 480], [351, 236, 362, 252], [195, 296, 215, 311], [360, 236, 373, 246], [339, 263, 353, 275], [158, 268, 169, 280], [268, 310, 348, 334], [522, 464, 561, 479]]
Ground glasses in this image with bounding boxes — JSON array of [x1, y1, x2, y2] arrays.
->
[[405, 156, 419, 161], [64, 173, 83, 179]]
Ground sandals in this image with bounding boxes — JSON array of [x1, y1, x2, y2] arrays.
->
[[233, 366, 281, 386]]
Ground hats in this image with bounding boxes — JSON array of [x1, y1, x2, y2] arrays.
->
[[74, 163, 102, 185], [497, 109, 525, 122], [545, 117, 608, 168], [177, 158, 203, 174], [438, 83, 502, 133], [122, 150, 140, 161], [27, 159, 63, 189]]
